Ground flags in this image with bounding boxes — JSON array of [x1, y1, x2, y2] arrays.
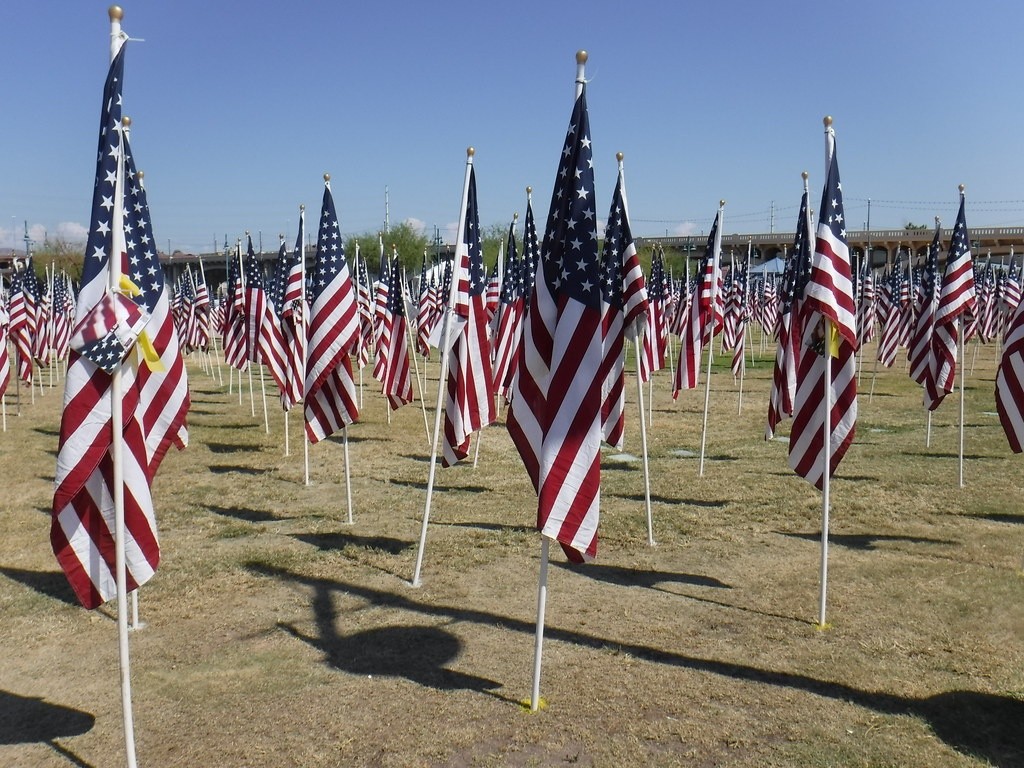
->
[[1, 163, 1023, 506], [301, 180, 362, 444], [786, 128, 859, 492], [50, 24, 191, 612], [505, 78, 603, 567], [440, 161, 496, 471]]
